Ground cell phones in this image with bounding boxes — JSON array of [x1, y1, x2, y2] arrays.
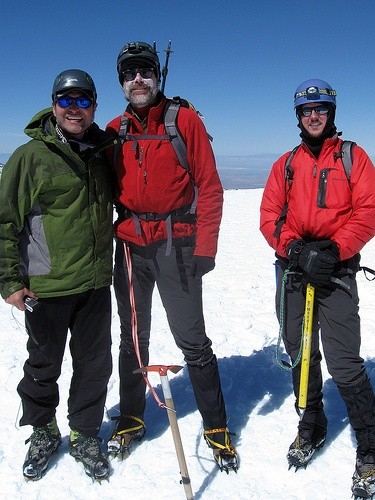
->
[[24, 296, 40, 312]]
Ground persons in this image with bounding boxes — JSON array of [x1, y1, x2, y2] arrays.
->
[[0, 69, 114, 485], [260, 79, 375, 500], [105, 41, 238, 475]]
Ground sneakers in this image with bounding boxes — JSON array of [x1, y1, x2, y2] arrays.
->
[[68, 428, 110, 485], [104, 418, 146, 462], [287, 433, 327, 471], [23, 417, 62, 482], [351, 456, 375, 500], [205, 431, 238, 472]]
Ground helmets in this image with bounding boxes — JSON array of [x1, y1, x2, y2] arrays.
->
[[293, 79, 336, 110], [116, 42, 161, 87], [52, 69, 97, 104]]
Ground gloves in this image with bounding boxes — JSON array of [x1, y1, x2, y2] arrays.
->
[[286, 237, 339, 281], [191, 255, 216, 279]]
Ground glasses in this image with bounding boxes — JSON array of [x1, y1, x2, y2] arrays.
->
[[56, 95, 92, 109], [122, 67, 155, 80], [297, 105, 329, 116]]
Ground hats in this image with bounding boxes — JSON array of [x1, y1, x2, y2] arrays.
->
[[56, 88, 93, 101]]
